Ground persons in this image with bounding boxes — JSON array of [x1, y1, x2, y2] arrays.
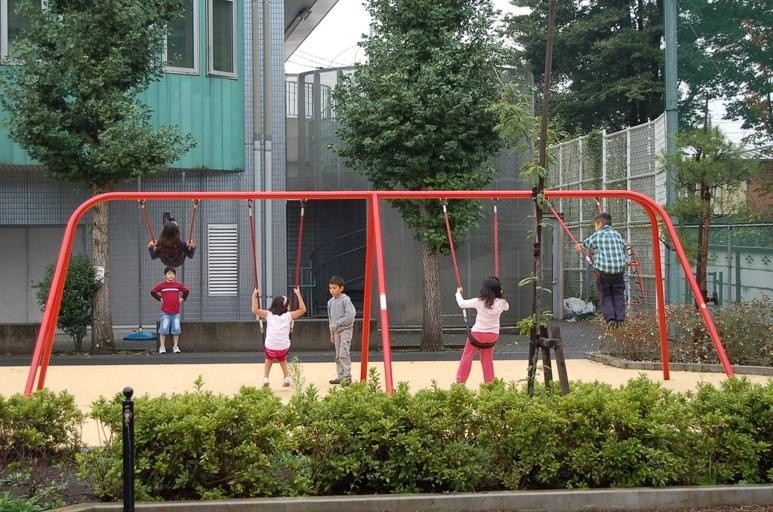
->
[[250, 285, 307, 388], [574, 213, 629, 330], [454, 275, 510, 387], [146, 210, 196, 268], [148, 266, 190, 355], [326, 275, 356, 387]]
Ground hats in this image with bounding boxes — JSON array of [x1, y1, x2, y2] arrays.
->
[[482, 276, 502, 292]]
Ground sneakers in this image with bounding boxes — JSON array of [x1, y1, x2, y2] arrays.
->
[[262, 377, 270, 387], [282, 377, 290, 387], [172, 345, 181, 354], [158, 345, 167, 355], [329, 379, 342, 384], [607, 320, 624, 331]]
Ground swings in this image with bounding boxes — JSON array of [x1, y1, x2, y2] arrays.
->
[[246, 198, 306, 359], [543, 198, 624, 283], [439, 197, 499, 348], [139, 197, 199, 267]]
